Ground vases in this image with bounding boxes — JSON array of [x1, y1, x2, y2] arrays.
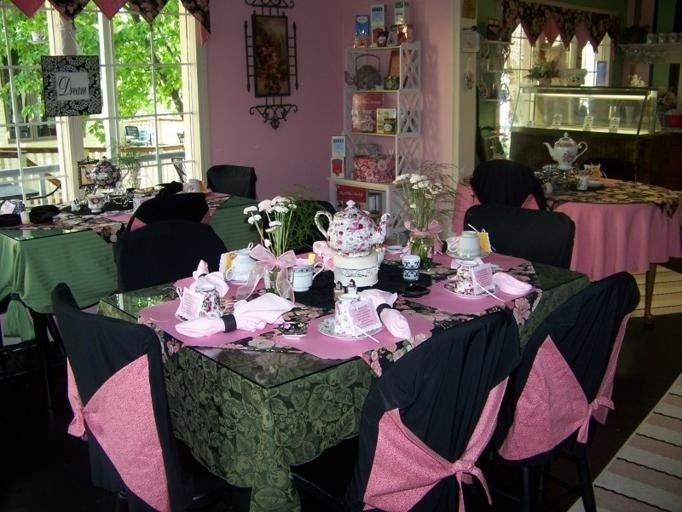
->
[[409, 224, 434, 273], [264, 259, 296, 308], [539, 78, 551, 88]]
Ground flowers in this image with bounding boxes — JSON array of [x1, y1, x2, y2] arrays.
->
[[655, 86, 678, 113], [524, 59, 565, 80], [244, 194, 297, 247], [393, 171, 446, 203]]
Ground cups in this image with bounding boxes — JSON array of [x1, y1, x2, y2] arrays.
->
[[87, 194, 105, 213], [133, 198, 144, 212], [402, 255, 420, 270], [403, 271, 419, 281], [195, 251, 256, 317], [188, 178, 200, 193], [577, 176, 589, 190], [454, 231, 481, 293], [290, 261, 362, 335]]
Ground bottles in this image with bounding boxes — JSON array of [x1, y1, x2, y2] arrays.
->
[[412, 236, 435, 270]]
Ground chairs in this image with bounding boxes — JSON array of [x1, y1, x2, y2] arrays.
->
[[54, 282, 231, 512], [205, 164, 260, 200], [285, 199, 337, 259], [293, 309, 522, 511], [462, 201, 573, 273], [117, 190, 230, 291], [491, 272, 639, 506]]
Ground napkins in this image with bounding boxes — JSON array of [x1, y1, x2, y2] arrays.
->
[[358, 287, 412, 338], [175, 298, 278, 345]]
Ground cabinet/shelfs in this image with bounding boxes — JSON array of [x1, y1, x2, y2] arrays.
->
[[328, 39, 423, 246], [511, 85, 657, 188]]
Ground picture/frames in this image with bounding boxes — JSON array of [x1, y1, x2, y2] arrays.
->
[[40, 54, 103, 117]]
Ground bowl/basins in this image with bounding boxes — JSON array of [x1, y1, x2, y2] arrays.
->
[[562, 68, 587, 86]]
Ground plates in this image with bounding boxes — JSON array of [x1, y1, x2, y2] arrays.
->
[[443, 280, 496, 299], [318, 317, 385, 341]]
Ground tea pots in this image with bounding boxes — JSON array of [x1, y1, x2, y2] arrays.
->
[[314, 198, 390, 256], [81, 156, 129, 189], [543, 132, 588, 170], [345, 53, 382, 89]]
